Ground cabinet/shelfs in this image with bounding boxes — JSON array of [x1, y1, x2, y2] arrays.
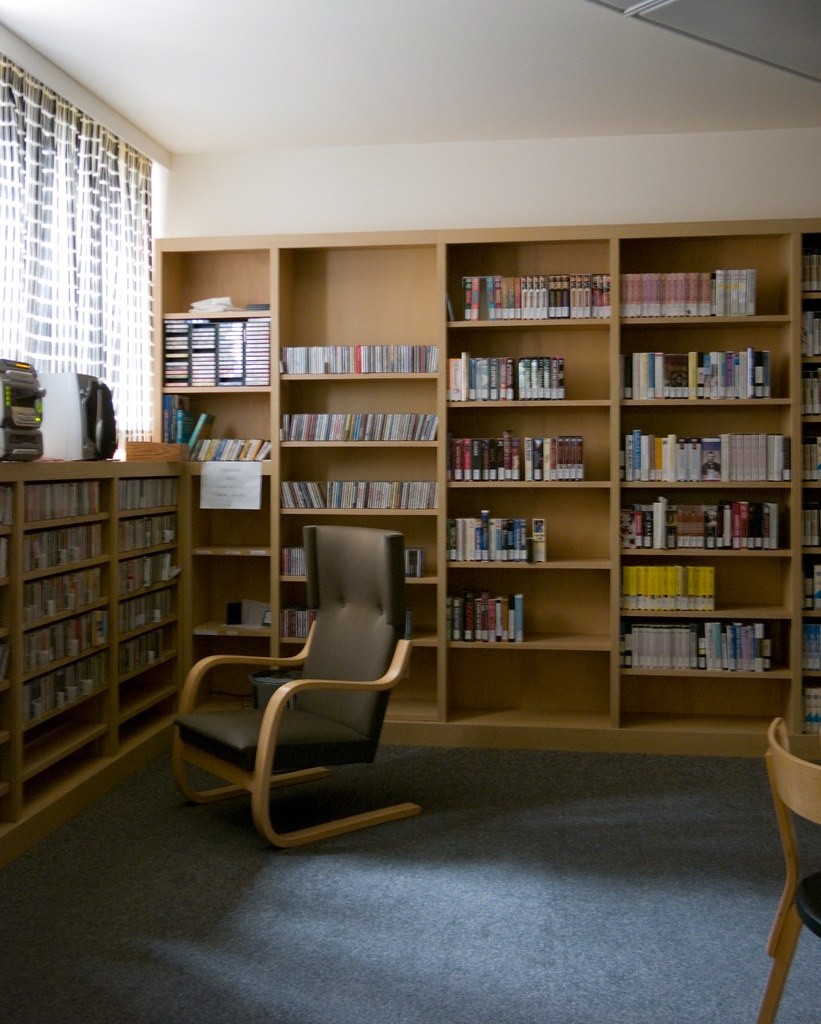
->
[[153, 218, 821, 760], [0, 461, 192, 870]]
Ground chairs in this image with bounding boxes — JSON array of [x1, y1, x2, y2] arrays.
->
[[756, 717, 821, 1024], [169, 524, 421, 846]]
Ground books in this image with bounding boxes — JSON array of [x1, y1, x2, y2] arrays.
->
[[619, 622, 771, 673], [282, 548, 307, 576], [404, 611, 412, 639], [446, 592, 524, 642], [800, 247, 821, 730], [620, 429, 790, 481], [0, 477, 182, 722], [618, 565, 714, 610], [461, 274, 611, 319], [280, 609, 313, 638], [445, 352, 567, 400], [281, 413, 438, 442], [404, 549, 425, 577], [280, 481, 439, 509], [446, 510, 543, 563], [162, 395, 272, 462], [620, 347, 771, 399], [621, 269, 757, 318], [280, 344, 439, 374], [448, 431, 585, 481], [162, 317, 270, 386], [620, 496, 779, 550]]
